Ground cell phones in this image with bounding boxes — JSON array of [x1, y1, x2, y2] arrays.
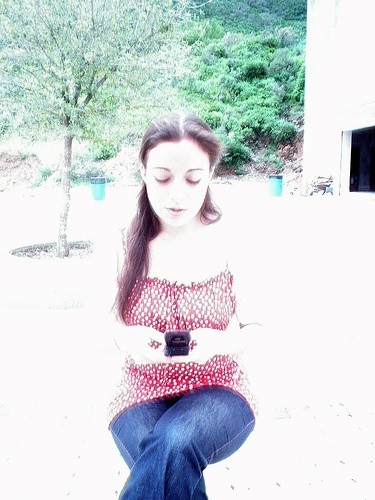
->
[[163, 328, 191, 357]]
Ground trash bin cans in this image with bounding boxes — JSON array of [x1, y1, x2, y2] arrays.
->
[[91, 176, 106, 202], [269, 174, 283, 197]]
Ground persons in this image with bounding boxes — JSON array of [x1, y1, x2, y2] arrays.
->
[[94, 110, 268, 500]]
